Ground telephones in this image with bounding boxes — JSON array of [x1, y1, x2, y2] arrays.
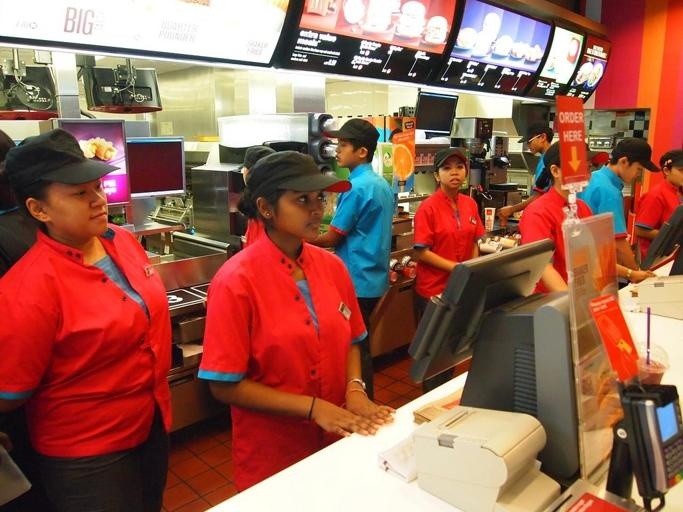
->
[[621, 384, 681, 510]]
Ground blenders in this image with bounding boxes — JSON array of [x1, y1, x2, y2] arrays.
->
[[450, 119, 495, 220]]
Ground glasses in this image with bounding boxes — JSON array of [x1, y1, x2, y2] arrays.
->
[[527, 134, 541, 143]]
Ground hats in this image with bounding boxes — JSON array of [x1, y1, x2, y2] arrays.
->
[[434, 148, 467, 171], [4, 129, 120, 190], [518, 123, 550, 143], [662, 150, 683, 166], [616, 137, 660, 172], [252, 151, 352, 193], [546, 140, 591, 171], [239, 145, 276, 170], [323, 118, 380, 142], [592, 152, 612, 165]]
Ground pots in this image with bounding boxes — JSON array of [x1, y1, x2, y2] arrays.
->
[[495, 155, 508, 166]]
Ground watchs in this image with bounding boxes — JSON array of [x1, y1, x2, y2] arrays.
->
[[627, 268, 632, 279]]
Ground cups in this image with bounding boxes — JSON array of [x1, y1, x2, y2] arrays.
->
[[499, 211, 509, 228], [636, 342, 668, 386], [484, 208, 496, 232]]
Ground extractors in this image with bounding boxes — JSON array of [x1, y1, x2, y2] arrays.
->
[[507, 138, 537, 176]]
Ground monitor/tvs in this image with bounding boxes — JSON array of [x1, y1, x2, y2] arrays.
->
[[81, 67, 163, 115], [640, 205, 683, 270], [123, 136, 187, 200], [413, 91, 459, 135], [0, 64, 58, 121], [512, 99, 551, 137], [407, 237, 556, 384]]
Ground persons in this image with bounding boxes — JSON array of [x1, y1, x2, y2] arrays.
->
[[635, 151, 683, 239], [2, 131, 45, 510], [0, 129, 173, 512], [576, 138, 661, 272], [311, 120, 397, 399], [237, 145, 277, 247], [198, 154, 392, 493], [413, 147, 487, 395], [519, 140, 656, 296], [497, 126, 554, 217]]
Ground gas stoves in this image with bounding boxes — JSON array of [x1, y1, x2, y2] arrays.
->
[[519, 184, 527, 192]]
